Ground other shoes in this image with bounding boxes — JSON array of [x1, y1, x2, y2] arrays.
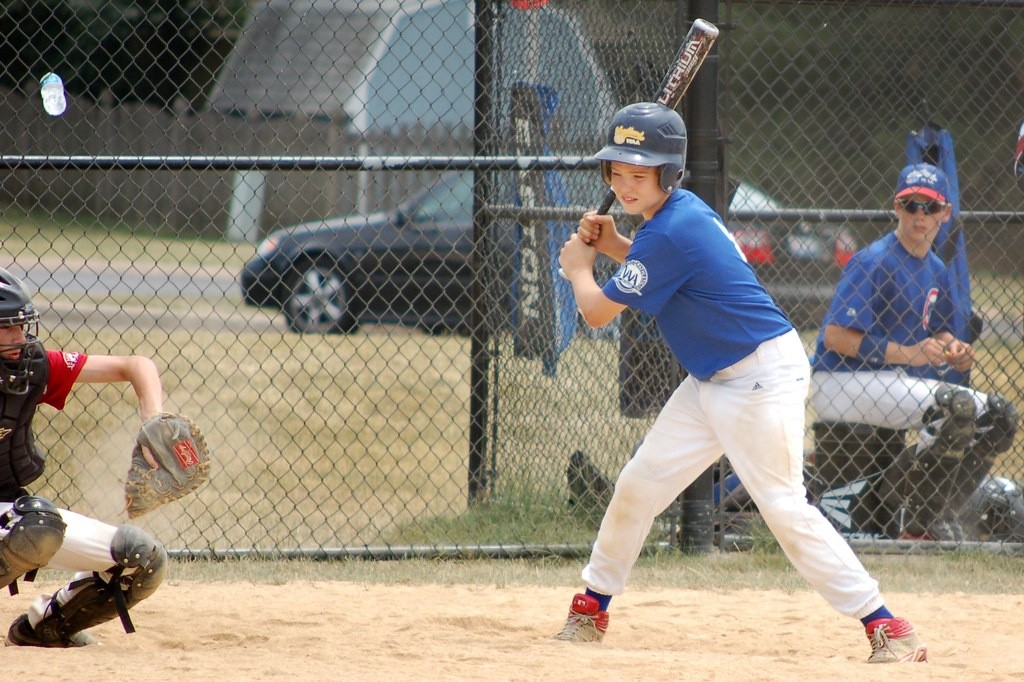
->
[[898, 516, 966, 553]]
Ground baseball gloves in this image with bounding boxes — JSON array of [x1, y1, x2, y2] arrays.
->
[[122, 413, 210, 519]]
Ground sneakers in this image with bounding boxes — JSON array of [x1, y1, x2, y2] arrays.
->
[[555, 593, 610, 644], [864, 618, 928, 664], [6, 613, 98, 648]]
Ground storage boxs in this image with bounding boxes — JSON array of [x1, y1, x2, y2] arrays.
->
[[814, 419, 908, 544]]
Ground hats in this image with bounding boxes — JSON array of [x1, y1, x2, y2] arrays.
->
[[896, 163, 950, 206]]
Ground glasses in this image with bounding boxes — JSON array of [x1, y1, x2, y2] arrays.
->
[[898, 197, 947, 215]]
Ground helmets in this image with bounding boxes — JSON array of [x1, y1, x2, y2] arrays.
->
[[0, 266, 40, 396], [593, 101, 687, 196]]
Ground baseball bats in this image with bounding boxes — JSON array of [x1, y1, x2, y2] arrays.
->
[[558, 19, 720, 289]]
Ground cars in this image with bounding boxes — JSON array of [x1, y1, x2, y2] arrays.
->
[[242, 170, 860, 342]]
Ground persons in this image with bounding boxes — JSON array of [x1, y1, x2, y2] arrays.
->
[[806, 160, 1017, 538], [1, 268, 175, 647], [553, 100, 928, 666]]
